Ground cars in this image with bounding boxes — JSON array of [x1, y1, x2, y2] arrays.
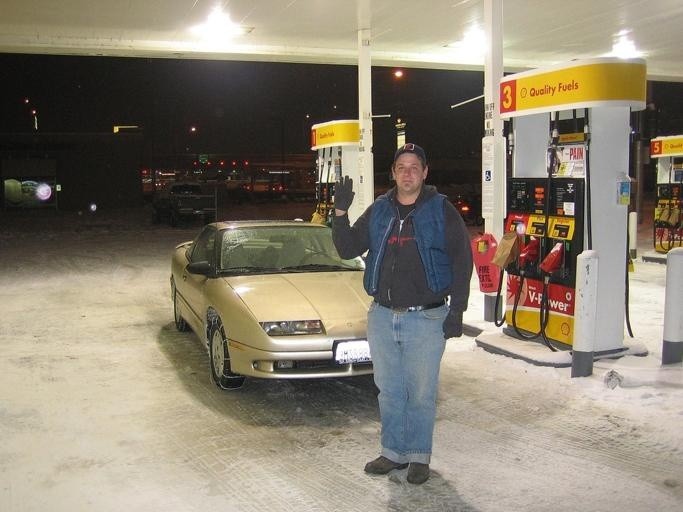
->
[[452, 191, 484, 226], [170, 219, 373, 391]]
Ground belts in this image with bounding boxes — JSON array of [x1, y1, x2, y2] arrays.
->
[[374, 299, 448, 312]]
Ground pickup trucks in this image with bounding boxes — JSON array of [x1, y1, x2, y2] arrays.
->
[[151, 181, 217, 227]]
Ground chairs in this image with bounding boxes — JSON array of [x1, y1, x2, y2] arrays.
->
[[222, 245, 251, 267], [278, 238, 312, 266]]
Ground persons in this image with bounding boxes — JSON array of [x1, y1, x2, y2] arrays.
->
[[333, 142, 474, 486]]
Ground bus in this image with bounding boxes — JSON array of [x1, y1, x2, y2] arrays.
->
[[136, 152, 317, 199]]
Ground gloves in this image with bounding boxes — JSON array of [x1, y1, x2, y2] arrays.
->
[[332, 174, 355, 211], [440, 307, 464, 339]]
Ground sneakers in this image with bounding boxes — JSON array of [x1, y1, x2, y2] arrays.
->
[[406, 461, 429, 484], [364, 456, 408, 475]]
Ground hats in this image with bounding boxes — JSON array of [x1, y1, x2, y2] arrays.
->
[[393, 143, 425, 165]]
